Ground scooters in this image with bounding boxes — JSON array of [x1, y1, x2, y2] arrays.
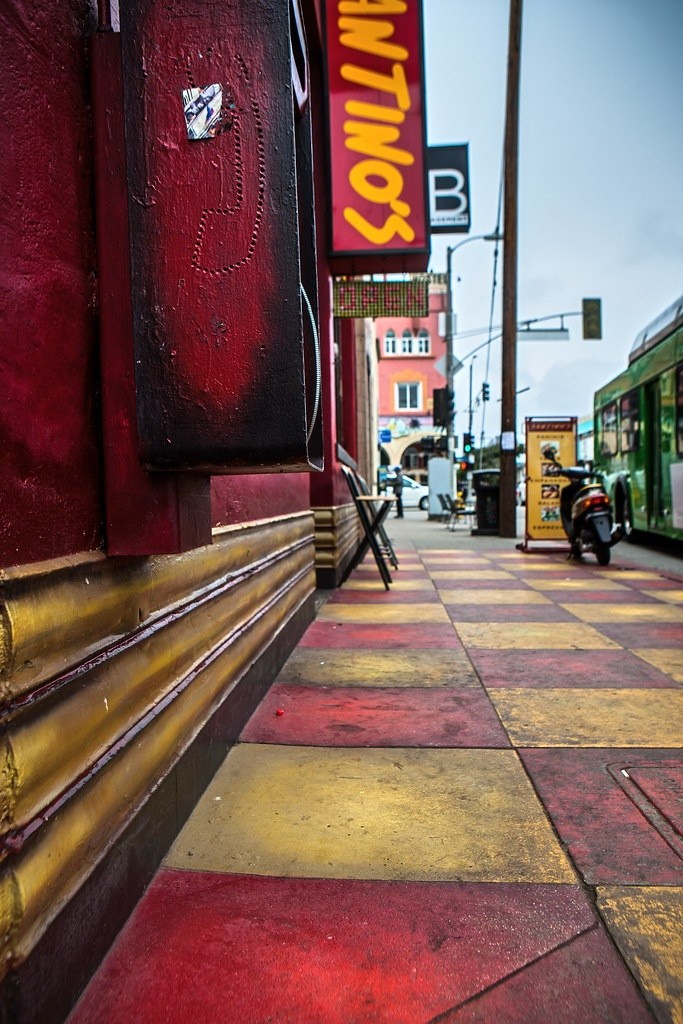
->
[[543, 448, 615, 566]]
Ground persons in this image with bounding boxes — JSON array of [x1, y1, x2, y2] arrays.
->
[[457, 483, 468, 519], [385, 467, 404, 518]]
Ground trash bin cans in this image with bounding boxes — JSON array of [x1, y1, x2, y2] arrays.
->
[[469, 468, 504, 536]]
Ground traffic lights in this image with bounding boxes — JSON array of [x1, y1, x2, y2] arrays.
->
[[482, 383, 490, 403], [461, 461, 469, 470], [463, 433, 472, 454], [433, 387, 454, 426]]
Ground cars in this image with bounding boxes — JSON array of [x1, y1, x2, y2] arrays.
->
[[387, 474, 431, 511]]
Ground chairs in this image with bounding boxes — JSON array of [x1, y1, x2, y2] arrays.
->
[[336, 465, 400, 591], [437, 493, 477, 531]]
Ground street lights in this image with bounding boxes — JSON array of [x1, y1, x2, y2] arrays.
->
[[446, 233, 505, 462]]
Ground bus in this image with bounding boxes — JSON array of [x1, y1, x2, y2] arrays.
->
[[592, 297, 683, 544]]
